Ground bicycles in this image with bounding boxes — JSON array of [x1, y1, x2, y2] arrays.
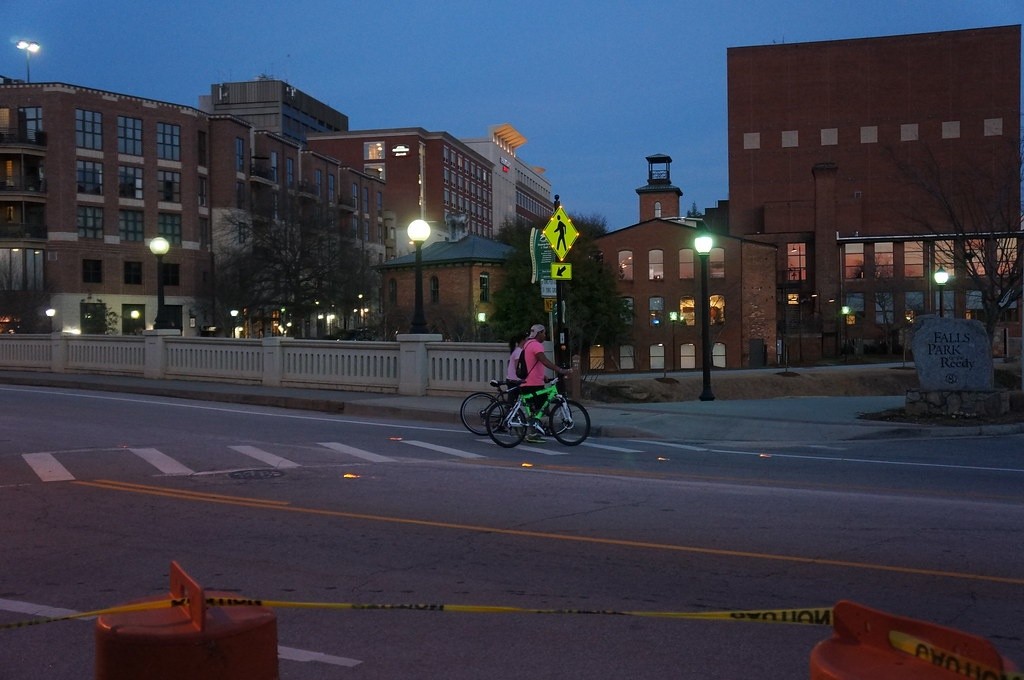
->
[[460, 378, 572, 436], [485, 375, 590, 448]]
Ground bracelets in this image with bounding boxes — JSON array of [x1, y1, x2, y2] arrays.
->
[[558, 368, 562, 373]]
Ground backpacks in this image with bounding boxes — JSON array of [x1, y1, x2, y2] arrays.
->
[[515, 341, 539, 380]]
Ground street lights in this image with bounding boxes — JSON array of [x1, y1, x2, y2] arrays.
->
[[842, 305, 852, 364], [131, 310, 139, 335], [46, 308, 55, 333], [329, 314, 334, 335], [150, 237, 174, 329], [799, 295, 817, 363], [406, 219, 431, 334], [934, 265, 949, 317], [230, 310, 238, 338], [820, 299, 835, 357], [694, 231, 716, 401]]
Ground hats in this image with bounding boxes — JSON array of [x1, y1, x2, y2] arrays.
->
[[528, 324, 545, 338]]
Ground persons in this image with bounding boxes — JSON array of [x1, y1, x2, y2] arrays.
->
[[521, 324, 575, 443], [494, 329, 528, 433]]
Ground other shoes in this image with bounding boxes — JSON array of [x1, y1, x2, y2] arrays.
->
[[527, 433, 547, 442]]
